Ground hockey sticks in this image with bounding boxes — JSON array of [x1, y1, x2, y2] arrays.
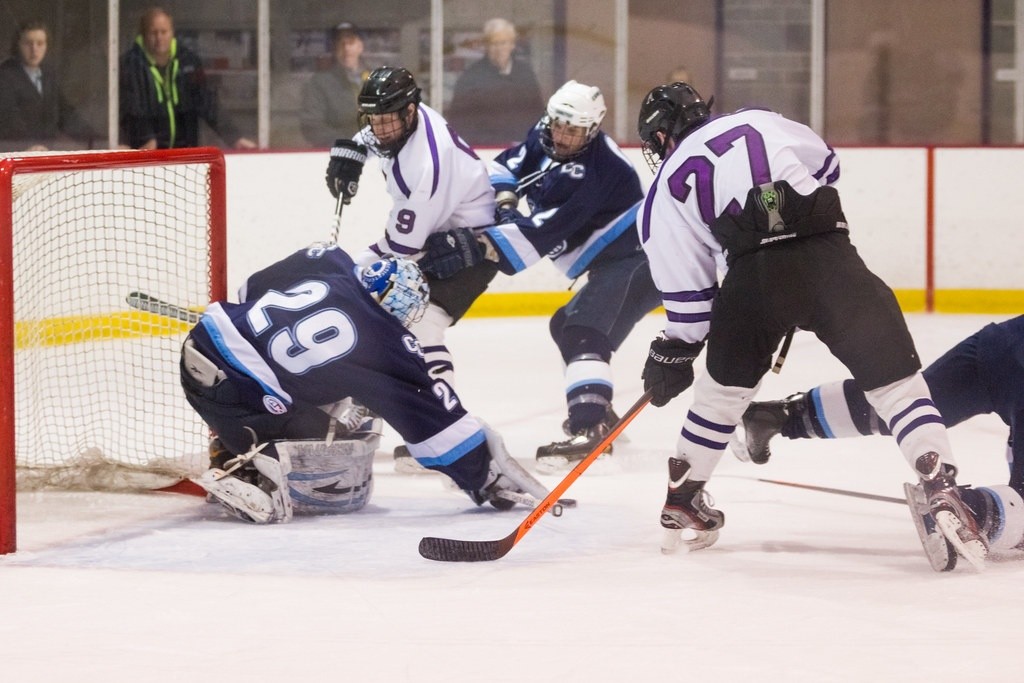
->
[[494, 488, 563, 517], [331, 189, 346, 241], [123, 290, 203, 323], [713, 471, 908, 507], [419, 387, 659, 564]]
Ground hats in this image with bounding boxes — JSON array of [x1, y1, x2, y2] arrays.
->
[[326, 22, 362, 52]]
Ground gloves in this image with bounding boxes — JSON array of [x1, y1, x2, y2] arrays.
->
[[640, 337, 705, 407], [417, 227, 484, 283], [326, 138, 368, 204], [494, 206, 524, 225]]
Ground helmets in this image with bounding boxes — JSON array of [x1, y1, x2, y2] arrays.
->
[[356, 66, 422, 159], [537, 80, 608, 162], [361, 253, 432, 330], [638, 81, 714, 175]]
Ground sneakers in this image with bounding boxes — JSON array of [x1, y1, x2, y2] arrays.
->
[[660, 457, 725, 554], [729, 399, 791, 465], [465, 464, 526, 511], [533, 418, 615, 476], [924, 473, 989, 566], [903, 481, 996, 574]]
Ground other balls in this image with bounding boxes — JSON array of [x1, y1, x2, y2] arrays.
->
[[557, 498, 576, 506]]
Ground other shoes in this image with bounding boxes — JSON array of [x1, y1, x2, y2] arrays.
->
[[206, 439, 236, 472]]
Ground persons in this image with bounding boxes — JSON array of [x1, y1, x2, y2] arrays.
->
[[300, 21, 373, 148], [119, 8, 258, 150], [742, 315, 1024, 571], [0, 19, 131, 151], [178, 242, 527, 523], [636, 82, 991, 564], [447, 18, 546, 144], [421, 80, 663, 461], [326, 65, 501, 462]]
